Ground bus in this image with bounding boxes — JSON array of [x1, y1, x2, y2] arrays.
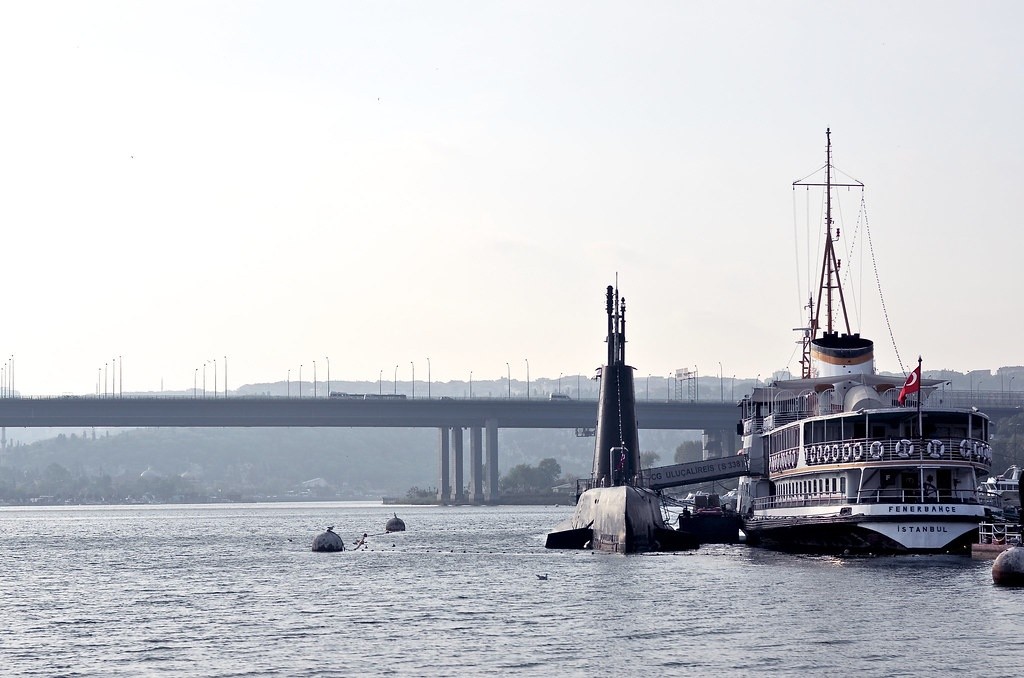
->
[[330, 391, 408, 400]]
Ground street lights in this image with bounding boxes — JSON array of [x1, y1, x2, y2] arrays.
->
[[0, 352, 1016, 407]]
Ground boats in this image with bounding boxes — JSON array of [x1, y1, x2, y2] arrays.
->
[[678, 495, 741, 543], [732, 125, 993, 560], [543, 272, 693, 551], [980, 463, 1021, 507]]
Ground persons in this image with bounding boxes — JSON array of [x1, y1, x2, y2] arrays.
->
[[1017, 499, 1024, 544], [986, 513, 995, 544]]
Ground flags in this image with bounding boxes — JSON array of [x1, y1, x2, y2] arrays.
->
[[898, 367, 919, 405]]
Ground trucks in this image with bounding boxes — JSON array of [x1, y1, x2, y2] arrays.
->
[[549, 392, 572, 401]]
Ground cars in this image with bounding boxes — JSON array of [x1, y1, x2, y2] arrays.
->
[[440, 396, 453, 401]]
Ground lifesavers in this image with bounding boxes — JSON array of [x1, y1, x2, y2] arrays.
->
[[852, 443, 863, 460], [895, 440, 914, 457], [770, 443, 852, 472], [959, 439, 993, 463], [870, 441, 884, 459], [926, 439, 944, 458]]
